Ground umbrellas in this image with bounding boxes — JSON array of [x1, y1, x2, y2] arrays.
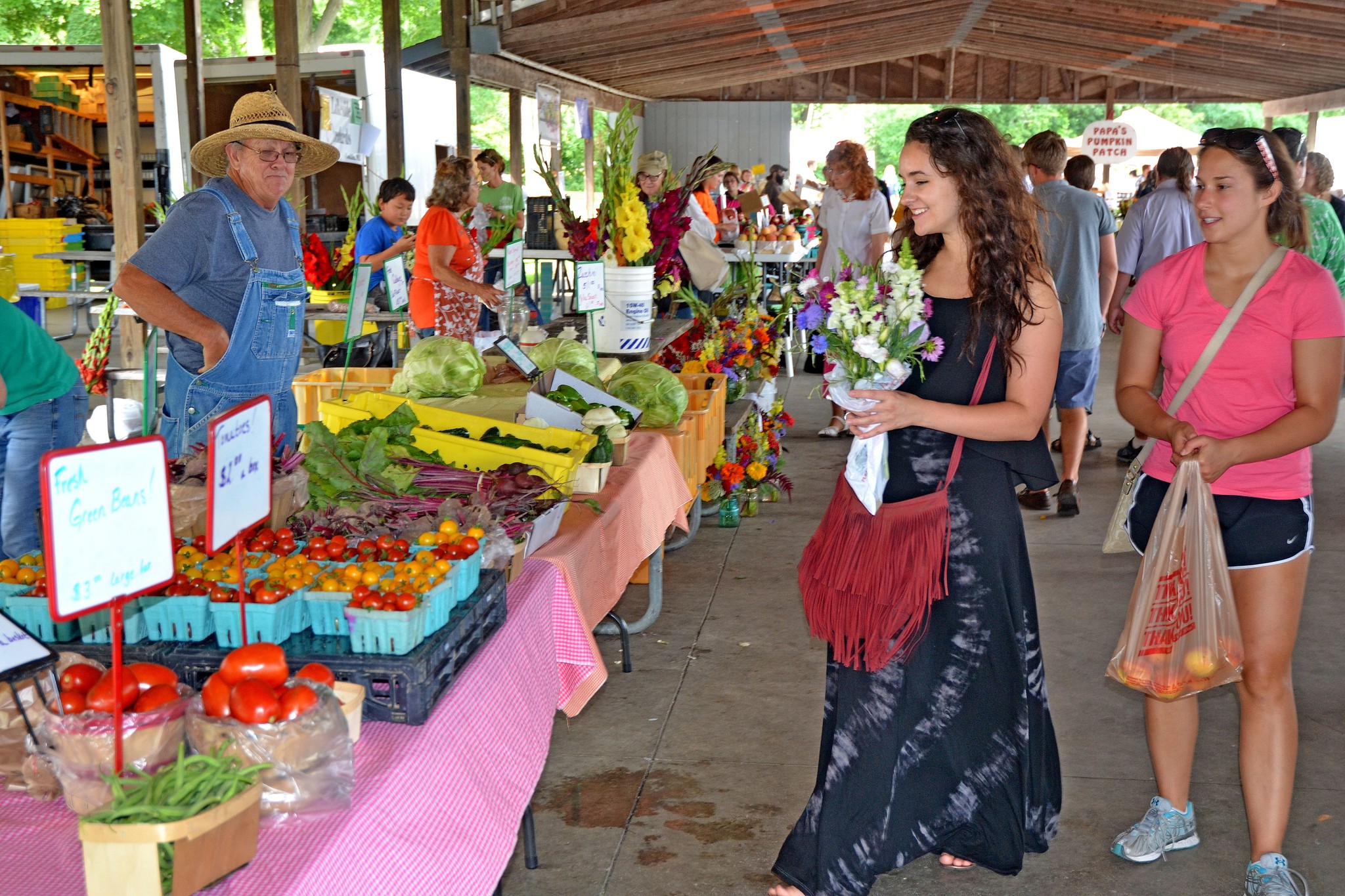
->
[[1063, 106, 1210, 163]]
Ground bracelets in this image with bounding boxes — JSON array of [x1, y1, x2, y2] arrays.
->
[[501, 214, 505, 220], [1103, 323, 1106, 334]]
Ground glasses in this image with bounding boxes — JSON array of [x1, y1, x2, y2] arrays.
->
[[231, 139, 304, 163], [1284, 127, 1305, 160], [777, 170, 785, 173], [826, 166, 852, 177], [468, 182, 483, 188], [637, 172, 664, 182], [725, 179, 737, 185], [476, 153, 496, 164]]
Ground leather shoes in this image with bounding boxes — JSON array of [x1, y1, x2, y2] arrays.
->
[[1018, 487, 1053, 511], [1056, 480, 1081, 515], [1117, 436, 1144, 462]]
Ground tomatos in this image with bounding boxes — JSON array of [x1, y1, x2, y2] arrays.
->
[[2, 509, 486, 646]]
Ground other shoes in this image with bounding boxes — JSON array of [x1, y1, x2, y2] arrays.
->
[[937, 853, 981, 869]]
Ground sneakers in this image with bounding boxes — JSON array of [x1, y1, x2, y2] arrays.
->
[[1110, 796, 1201, 863], [1245, 853, 1299, 896]]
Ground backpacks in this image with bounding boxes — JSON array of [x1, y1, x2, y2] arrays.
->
[[799, 462, 951, 674]]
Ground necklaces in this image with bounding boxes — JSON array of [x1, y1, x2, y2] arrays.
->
[[837, 190, 856, 202]]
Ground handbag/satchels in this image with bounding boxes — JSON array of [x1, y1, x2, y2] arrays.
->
[[678, 230, 731, 292], [1102, 460, 1143, 555], [1098, 460, 1246, 703]]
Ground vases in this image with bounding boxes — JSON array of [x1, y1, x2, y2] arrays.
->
[[675, 277, 799, 528]]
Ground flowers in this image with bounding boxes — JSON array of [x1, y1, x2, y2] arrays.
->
[[781, 236, 954, 516]]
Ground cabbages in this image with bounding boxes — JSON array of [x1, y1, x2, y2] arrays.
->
[[406, 324, 486, 405], [538, 363, 604, 392], [390, 373, 408, 395], [605, 359, 691, 431], [523, 335, 598, 374]]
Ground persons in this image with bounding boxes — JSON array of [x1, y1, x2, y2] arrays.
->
[[765, 106, 1063, 896], [759, 164, 809, 213], [0, 297, 89, 560], [723, 171, 745, 212], [1113, 126, 1344, 895], [719, 165, 740, 194], [112, 93, 339, 455], [1006, 127, 1344, 297], [469, 149, 525, 332], [1016, 130, 1118, 516], [355, 177, 417, 311], [808, 140, 889, 437], [737, 170, 751, 189], [1051, 154, 1102, 451], [802, 160, 903, 231], [1107, 147, 1205, 465], [690, 154, 737, 243], [627, 151, 716, 318], [408, 156, 528, 345]]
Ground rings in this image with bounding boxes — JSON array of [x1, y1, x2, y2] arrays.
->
[[844, 412, 851, 420]]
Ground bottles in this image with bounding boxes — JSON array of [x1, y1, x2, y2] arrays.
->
[[498, 296, 530, 346]]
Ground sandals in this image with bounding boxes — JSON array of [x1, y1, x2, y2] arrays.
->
[[1050, 429, 1102, 453], [818, 413, 854, 440]]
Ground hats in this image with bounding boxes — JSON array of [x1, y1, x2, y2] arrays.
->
[[770, 164, 788, 173], [634, 151, 668, 176], [189, 85, 340, 179]]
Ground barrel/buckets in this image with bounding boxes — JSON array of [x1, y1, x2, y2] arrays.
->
[[586, 266, 656, 354]]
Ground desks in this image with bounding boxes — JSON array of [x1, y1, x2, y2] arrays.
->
[[0, 313, 708, 892], [725, 236, 826, 311]]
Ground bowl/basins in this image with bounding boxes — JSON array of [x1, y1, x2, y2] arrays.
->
[[557, 326, 580, 340], [519, 325, 545, 354]]
